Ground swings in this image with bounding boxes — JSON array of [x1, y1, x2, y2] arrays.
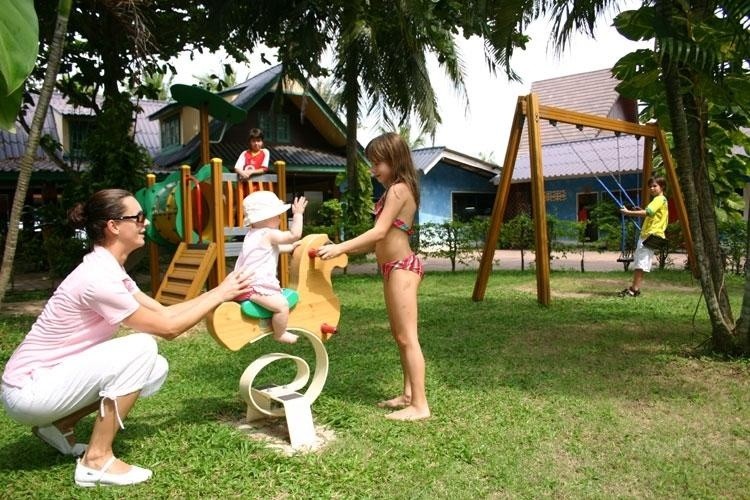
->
[[554, 125, 671, 262]]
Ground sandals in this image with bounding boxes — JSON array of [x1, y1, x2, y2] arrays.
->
[[618, 286, 640, 297]]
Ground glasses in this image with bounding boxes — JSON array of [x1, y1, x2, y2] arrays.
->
[[109, 211, 145, 223]]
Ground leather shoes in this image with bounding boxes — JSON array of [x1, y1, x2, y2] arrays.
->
[[31, 424, 88, 456], [74, 455, 152, 488]]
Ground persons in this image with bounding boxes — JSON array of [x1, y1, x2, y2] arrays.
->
[[317, 132, 431, 421], [232, 128, 270, 242], [1, 188, 257, 487], [618, 176, 667, 296], [234, 190, 309, 345]]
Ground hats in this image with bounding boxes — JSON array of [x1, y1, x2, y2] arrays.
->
[[242, 190, 291, 227]]
